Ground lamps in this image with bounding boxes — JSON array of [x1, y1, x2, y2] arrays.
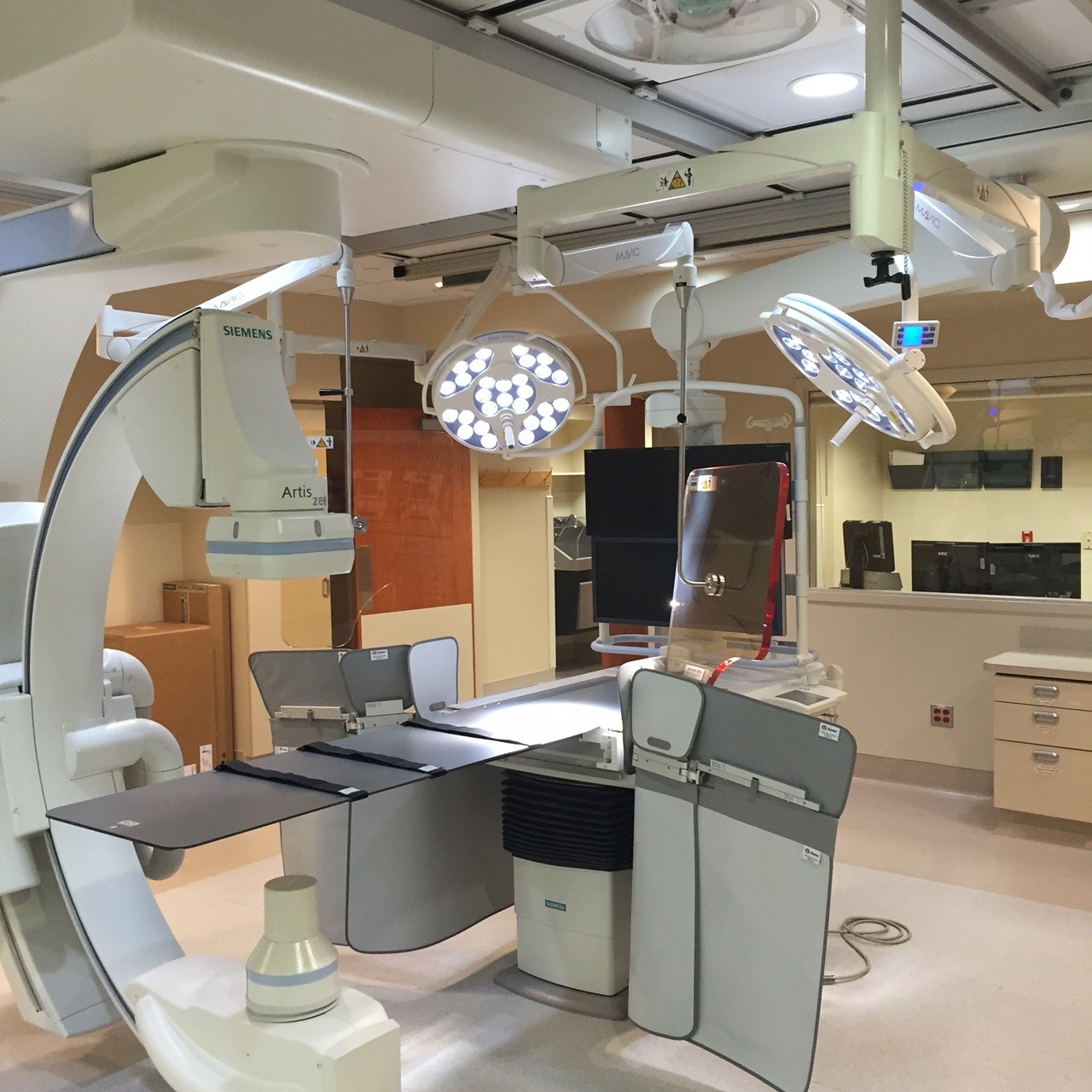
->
[[759, 291, 957, 449], [421, 328, 588, 455]]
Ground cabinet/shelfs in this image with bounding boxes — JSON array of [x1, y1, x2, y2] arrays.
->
[[991, 673, 1091, 823]]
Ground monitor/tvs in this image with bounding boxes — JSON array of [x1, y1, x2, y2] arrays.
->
[[584, 442, 794, 541], [588, 536, 787, 638], [987, 542, 1081, 601], [842, 520, 895, 573], [911, 540, 989, 595]]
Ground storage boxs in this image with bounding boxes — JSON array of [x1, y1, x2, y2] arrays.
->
[[104, 580, 236, 778]]
